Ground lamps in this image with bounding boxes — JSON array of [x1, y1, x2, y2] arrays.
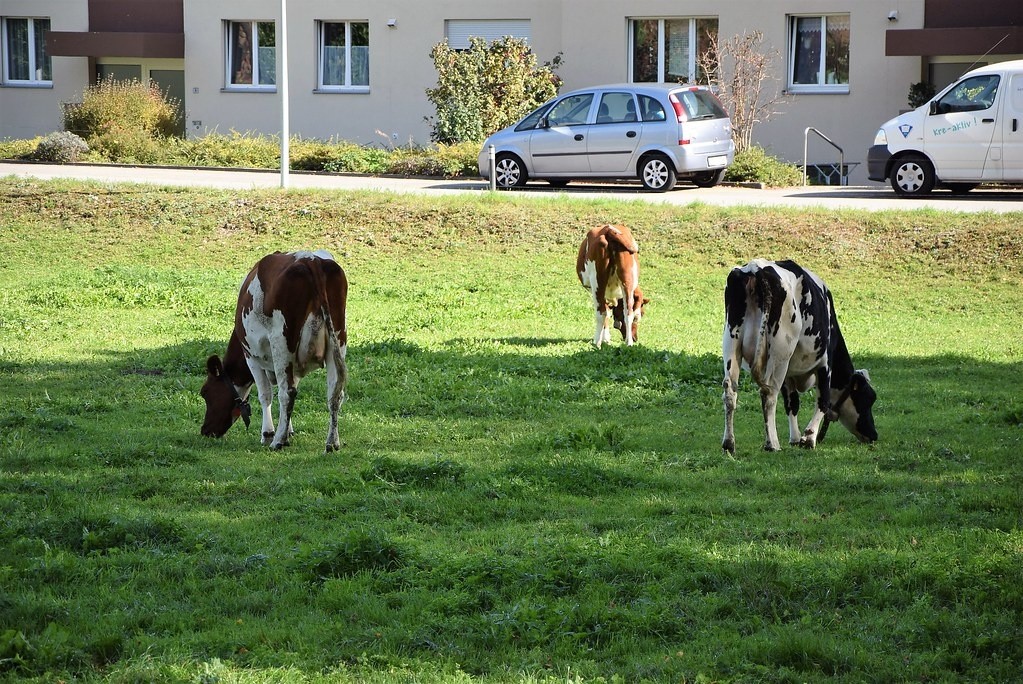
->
[[887, 10, 898, 21], [387, 18, 397, 26]]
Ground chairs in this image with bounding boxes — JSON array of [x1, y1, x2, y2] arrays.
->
[[646, 99, 661, 120], [597, 103, 612, 123], [625, 99, 644, 121]]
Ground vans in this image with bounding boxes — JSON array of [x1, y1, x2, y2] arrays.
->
[[867, 57, 1023, 200]]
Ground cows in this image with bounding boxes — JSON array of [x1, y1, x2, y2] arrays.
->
[[720, 258, 879, 455], [575, 223, 652, 350], [200, 248, 349, 454]]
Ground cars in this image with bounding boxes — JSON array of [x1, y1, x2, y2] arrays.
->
[[476, 80, 735, 194]]
